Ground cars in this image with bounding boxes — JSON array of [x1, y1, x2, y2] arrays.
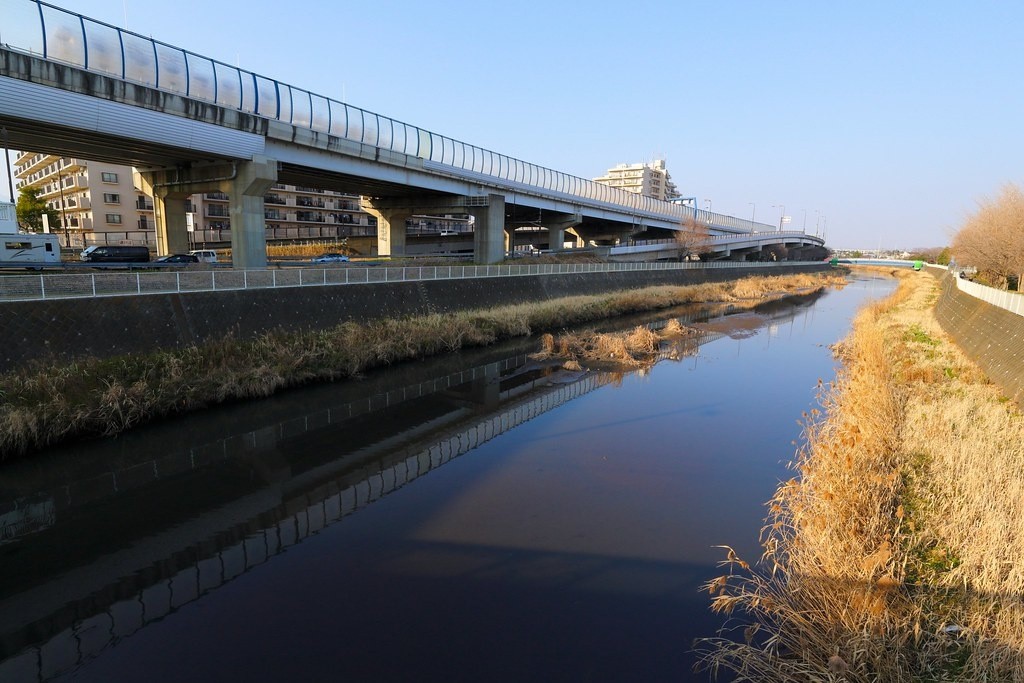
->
[[152, 254, 199, 271], [311, 253, 349, 262]]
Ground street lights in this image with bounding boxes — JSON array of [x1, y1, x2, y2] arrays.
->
[[58, 157, 70, 246], [749, 202, 755, 233], [801, 209, 827, 241], [772, 206, 785, 231], [705, 199, 711, 236]]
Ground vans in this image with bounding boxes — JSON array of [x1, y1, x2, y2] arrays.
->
[[189, 250, 218, 263], [79, 245, 149, 270], [441, 230, 457, 235]]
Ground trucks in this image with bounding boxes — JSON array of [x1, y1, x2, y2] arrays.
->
[[0, 234, 61, 270]]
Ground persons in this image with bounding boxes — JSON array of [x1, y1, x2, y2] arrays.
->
[[968, 272, 974, 282], [959, 270, 965, 278]]
